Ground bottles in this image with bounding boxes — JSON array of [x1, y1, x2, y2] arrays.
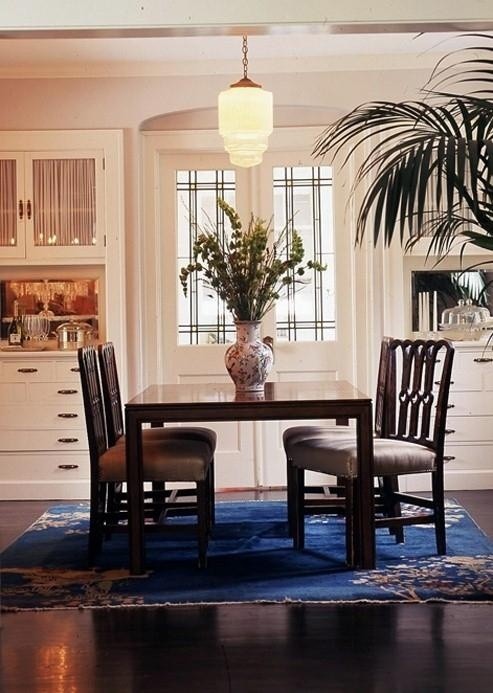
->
[[8, 300, 22, 345]]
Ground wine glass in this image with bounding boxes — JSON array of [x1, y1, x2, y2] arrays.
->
[[20, 313, 50, 346]]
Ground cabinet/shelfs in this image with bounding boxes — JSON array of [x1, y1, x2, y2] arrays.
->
[[392, 347, 493, 493], [2, 130, 123, 264], [1, 359, 91, 501]]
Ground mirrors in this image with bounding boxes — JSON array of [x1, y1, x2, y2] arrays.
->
[[411, 270, 493, 332]]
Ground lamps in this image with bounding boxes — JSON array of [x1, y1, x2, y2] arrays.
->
[[215, 34, 275, 169]]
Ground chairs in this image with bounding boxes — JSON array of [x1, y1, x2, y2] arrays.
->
[[98, 342, 218, 542], [77, 344, 213, 570], [282, 337, 453, 568]]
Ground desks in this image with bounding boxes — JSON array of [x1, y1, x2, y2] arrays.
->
[[124, 379, 376, 577]]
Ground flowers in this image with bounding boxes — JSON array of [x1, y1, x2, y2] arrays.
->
[[168, 194, 328, 324]]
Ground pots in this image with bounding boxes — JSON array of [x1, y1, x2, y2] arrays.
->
[[51, 320, 96, 351]]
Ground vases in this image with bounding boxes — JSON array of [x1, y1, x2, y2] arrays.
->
[[222, 321, 273, 392]]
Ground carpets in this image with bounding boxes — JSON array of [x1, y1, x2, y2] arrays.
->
[[1, 496, 493, 611]]
